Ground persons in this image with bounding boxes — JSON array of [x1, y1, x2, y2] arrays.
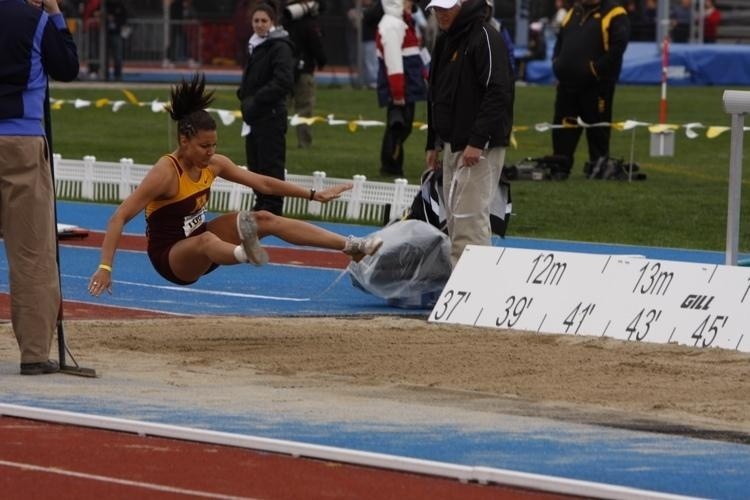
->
[[0, 1, 80, 376]]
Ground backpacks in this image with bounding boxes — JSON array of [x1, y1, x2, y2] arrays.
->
[[275, 38, 308, 108]]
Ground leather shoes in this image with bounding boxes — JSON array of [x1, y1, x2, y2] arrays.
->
[[19, 358, 60, 376]]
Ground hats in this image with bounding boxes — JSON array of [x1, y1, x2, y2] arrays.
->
[[424, 0, 458, 12]]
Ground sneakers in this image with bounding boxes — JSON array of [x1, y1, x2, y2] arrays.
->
[[344, 235, 384, 264], [237, 210, 270, 267]]
